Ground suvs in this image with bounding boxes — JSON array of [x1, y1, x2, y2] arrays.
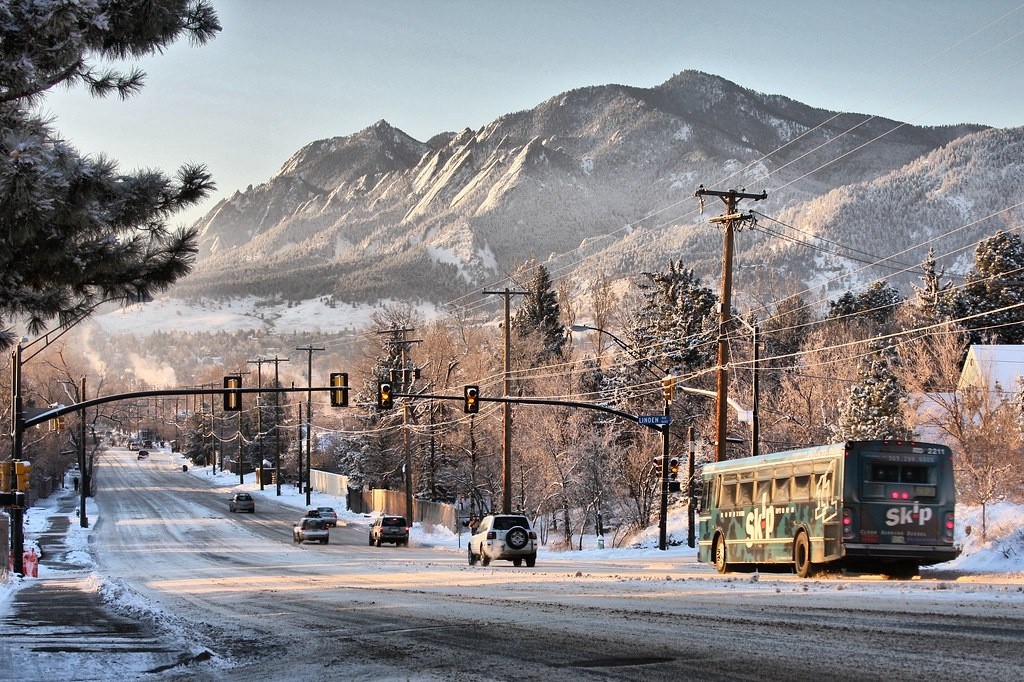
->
[[368, 514, 410, 548], [466, 512, 538, 568]]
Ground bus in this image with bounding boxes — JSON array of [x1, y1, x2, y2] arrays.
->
[[688, 436, 958, 579]]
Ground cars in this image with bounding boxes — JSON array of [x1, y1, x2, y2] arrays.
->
[[291, 506, 338, 544], [228, 493, 256, 513], [105, 431, 153, 460]]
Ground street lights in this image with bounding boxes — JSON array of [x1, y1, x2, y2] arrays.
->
[[569, 323, 670, 550], [12, 293, 155, 577]]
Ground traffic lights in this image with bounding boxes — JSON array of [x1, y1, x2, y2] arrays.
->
[[15, 460, 31, 492], [54, 402, 65, 431], [668, 481, 681, 492], [668, 455, 679, 479], [652, 456, 662, 478], [464, 385, 479, 414], [377, 380, 394, 410], [661, 376, 676, 401]]
